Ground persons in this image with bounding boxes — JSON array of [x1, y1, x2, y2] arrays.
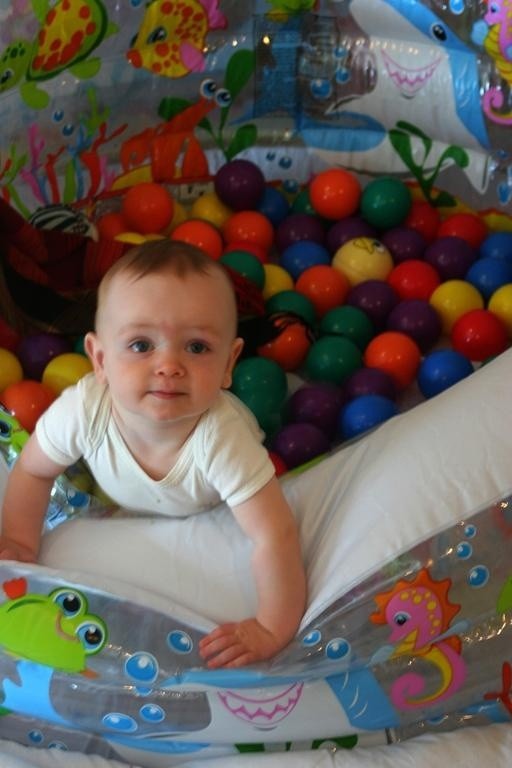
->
[[2, 196, 81, 303], [1, 238, 306, 672]]
